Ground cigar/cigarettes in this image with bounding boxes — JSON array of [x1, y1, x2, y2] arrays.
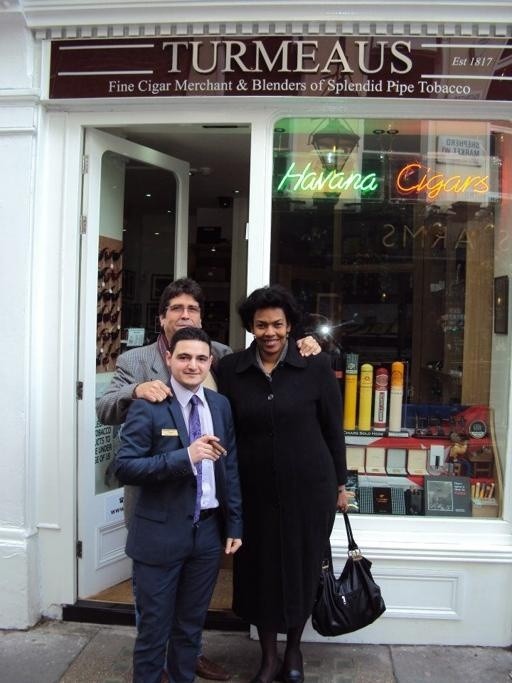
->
[[204, 433, 227, 456]]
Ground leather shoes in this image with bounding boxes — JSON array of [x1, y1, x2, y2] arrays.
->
[[196, 656, 230, 680], [284, 649, 302, 683]]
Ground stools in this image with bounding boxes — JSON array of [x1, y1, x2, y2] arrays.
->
[[467, 453, 493, 478]]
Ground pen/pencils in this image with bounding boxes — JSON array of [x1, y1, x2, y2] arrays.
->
[[471, 482, 495, 500]]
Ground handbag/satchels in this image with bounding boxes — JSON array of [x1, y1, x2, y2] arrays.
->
[[312, 555, 385, 636]]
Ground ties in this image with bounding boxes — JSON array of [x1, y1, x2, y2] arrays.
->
[[189, 396, 202, 523]]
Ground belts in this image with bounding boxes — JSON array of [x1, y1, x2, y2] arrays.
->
[[201, 509, 214, 520]]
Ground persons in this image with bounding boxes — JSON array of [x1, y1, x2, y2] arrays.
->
[[113, 325, 243, 683], [296, 307, 344, 361], [96, 277, 322, 683], [211, 287, 347, 683]]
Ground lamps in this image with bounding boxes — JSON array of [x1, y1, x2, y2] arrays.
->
[[308, 119, 361, 177]]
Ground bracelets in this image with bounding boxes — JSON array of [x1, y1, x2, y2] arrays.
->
[[338, 488, 345, 493]]
